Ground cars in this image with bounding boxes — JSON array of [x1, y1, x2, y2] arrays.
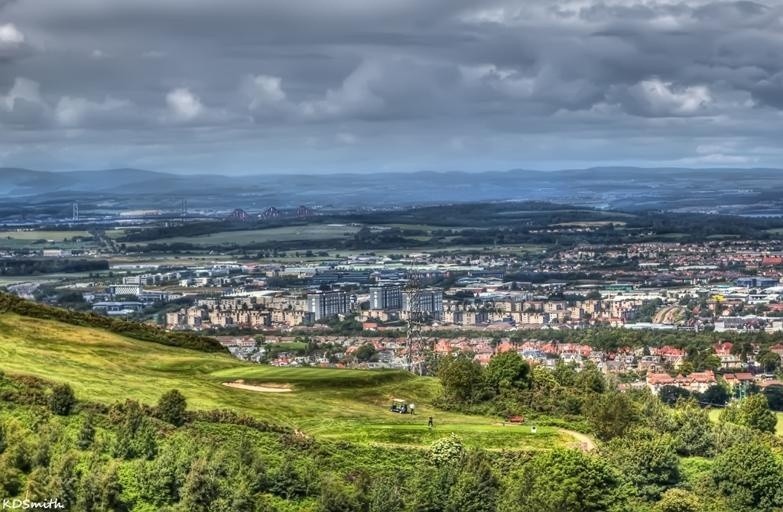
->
[[390, 398, 407, 413]]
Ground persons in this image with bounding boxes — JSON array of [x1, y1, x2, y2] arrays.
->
[[410, 402, 415, 414], [531, 426, 537, 433], [428, 415, 432, 426]]
